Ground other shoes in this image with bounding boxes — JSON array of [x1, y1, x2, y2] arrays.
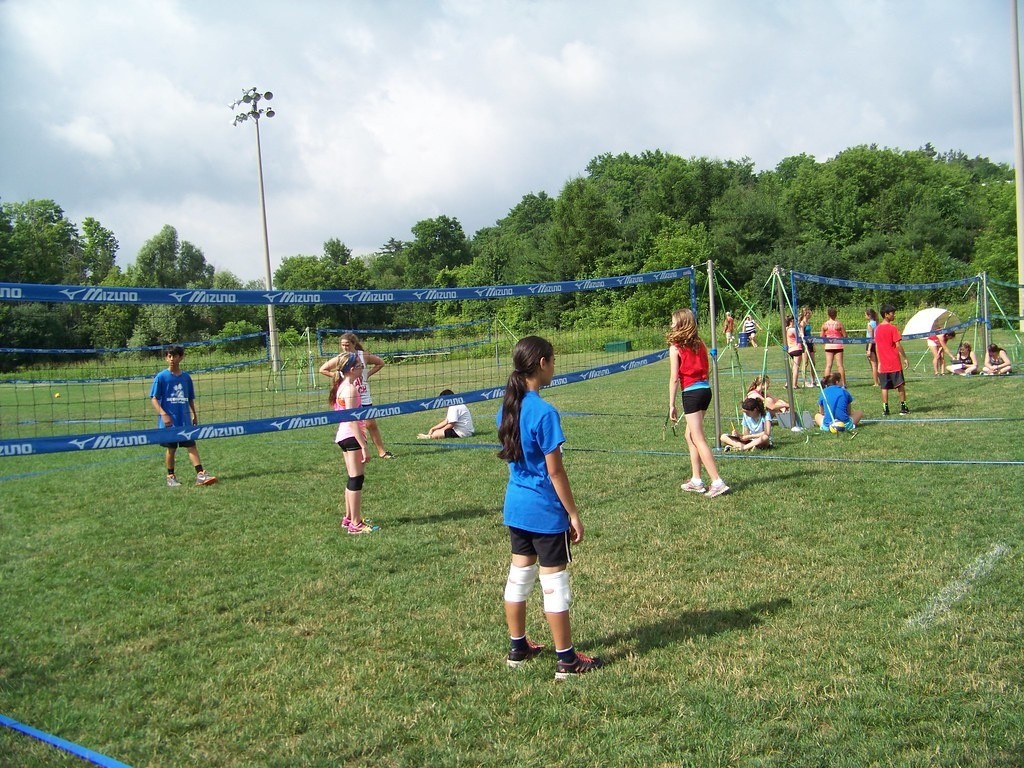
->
[[747, 446, 756, 453], [980, 372, 988, 375], [1004, 371, 1010, 374], [783, 386, 787, 390], [842, 385, 847, 389], [793, 385, 798, 389], [935, 373, 946, 377], [801, 381, 816, 388], [724, 445, 731, 455]]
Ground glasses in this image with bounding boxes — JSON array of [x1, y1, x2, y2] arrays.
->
[[354, 363, 364, 369]]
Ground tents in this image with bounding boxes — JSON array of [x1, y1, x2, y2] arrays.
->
[[902, 307, 963, 340]]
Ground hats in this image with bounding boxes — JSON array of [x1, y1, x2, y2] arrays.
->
[[726, 312, 730, 315]]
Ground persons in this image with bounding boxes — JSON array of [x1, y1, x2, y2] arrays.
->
[[494, 336, 603, 679], [319, 334, 399, 533], [416, 389, 475, 440], [719, 304, 1012, 454], [148, 346, 216, 485], [667, 309, 730, 498]]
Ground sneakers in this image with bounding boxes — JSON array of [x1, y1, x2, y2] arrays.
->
[[554, 653, 602, 680], [681, 480, 706, 492], [341, 516, 381, 534], [416, 433, 431, 439], [507, 645, 543, 667], [378, 452, 398, 459], [704, 484, 729, 498], [167, 474, 182, 486], [899, 409, 910, 415], [882, 409, 891, 417], [196, 470, 217, 485]]
